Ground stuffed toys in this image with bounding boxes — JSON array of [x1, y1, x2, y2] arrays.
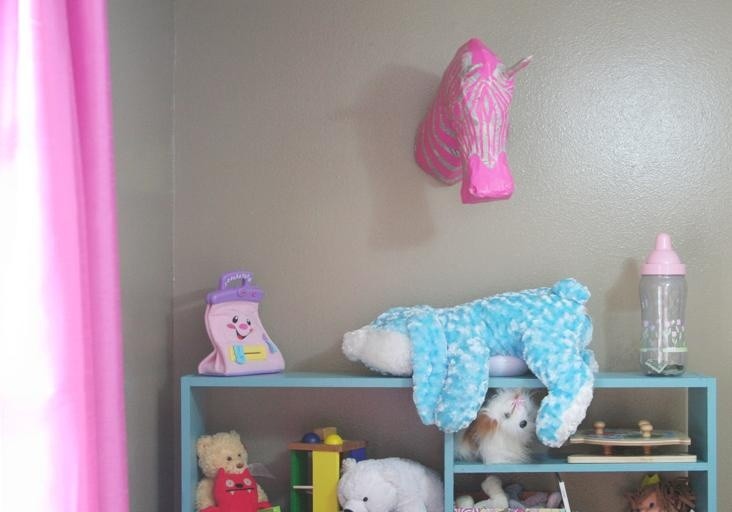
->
[[342, 277, 600, 449], [624, 473, 696, 512], [194, 428, 270, 512], [336, 455, 444, 512], [454, 475, 561, 508], [455, 388, 539, 464]]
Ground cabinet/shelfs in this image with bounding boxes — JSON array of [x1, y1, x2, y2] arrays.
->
[[181, 372, 717, 512]]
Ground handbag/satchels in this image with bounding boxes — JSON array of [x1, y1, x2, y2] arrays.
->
[[196, 270, 287, 377]]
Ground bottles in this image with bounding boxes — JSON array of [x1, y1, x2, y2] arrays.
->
[[637, 231, 688, 377]]
